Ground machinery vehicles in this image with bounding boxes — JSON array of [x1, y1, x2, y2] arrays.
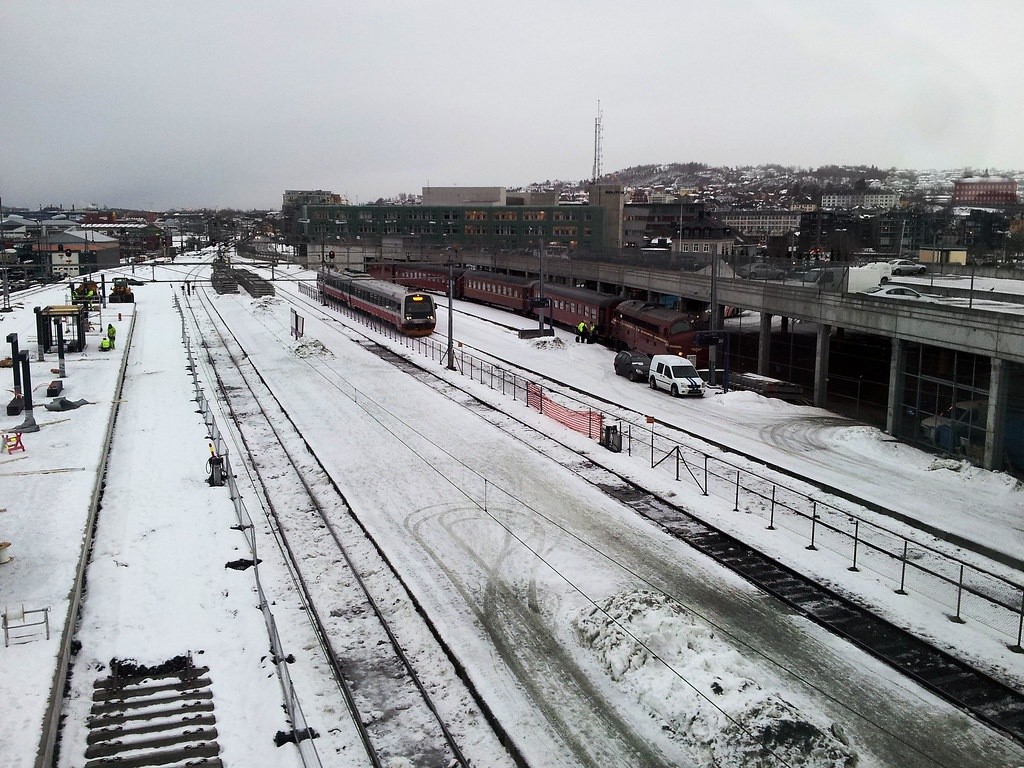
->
[[72, 277, 134, 303]]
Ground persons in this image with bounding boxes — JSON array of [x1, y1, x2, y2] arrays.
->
[[577, 319, 588, 343], [589, 320, 597, 334], [85, 289, 93, 310], [101, 337, 110, 350], [107, 324, 116, 348]]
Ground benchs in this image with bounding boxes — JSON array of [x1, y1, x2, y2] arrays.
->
[[47, 380, 62, 397], [68, 340, 76, 352]]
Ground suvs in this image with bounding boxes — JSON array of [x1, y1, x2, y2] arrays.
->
[[614, 350, 651, 382]]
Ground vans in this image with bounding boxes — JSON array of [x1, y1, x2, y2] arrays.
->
[[648, 354, 705, 397]]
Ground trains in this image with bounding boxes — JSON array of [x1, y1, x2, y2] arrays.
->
[[365, 259, 708, 369], [317, 263, 437, 337]]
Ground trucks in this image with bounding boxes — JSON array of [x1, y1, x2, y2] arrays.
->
[[786, 262, 891, 292]]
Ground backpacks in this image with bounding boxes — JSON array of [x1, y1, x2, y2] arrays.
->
[[583, 323, 587, 333]]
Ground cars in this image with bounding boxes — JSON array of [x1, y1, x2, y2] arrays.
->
[[737, 263, 785, 280], [856, 284, 939, 304], [888, 259, 927, 275]]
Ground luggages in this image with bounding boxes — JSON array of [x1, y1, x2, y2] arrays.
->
[[576, 335, 579, 342], [587, 332, 595, 344]]
